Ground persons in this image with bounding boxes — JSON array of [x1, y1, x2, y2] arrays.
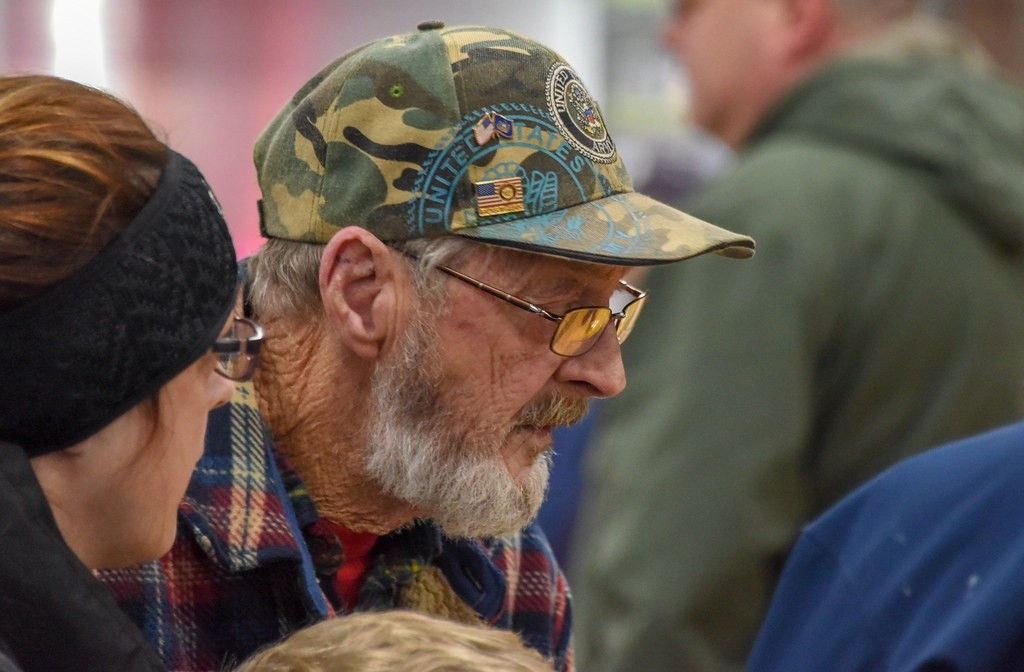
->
[[236, 610, 570, 672], [0, 72, 267, 672], [573, 1, 1024, 672], [96, 21, 754, 672], [745, 422, 1024, 672]]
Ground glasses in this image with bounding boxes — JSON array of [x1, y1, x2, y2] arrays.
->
[[209, 316, 266, 381], [381, 241, 650, 356]]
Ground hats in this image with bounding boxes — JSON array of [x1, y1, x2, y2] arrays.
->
[[254, 21, 755, 265]]
